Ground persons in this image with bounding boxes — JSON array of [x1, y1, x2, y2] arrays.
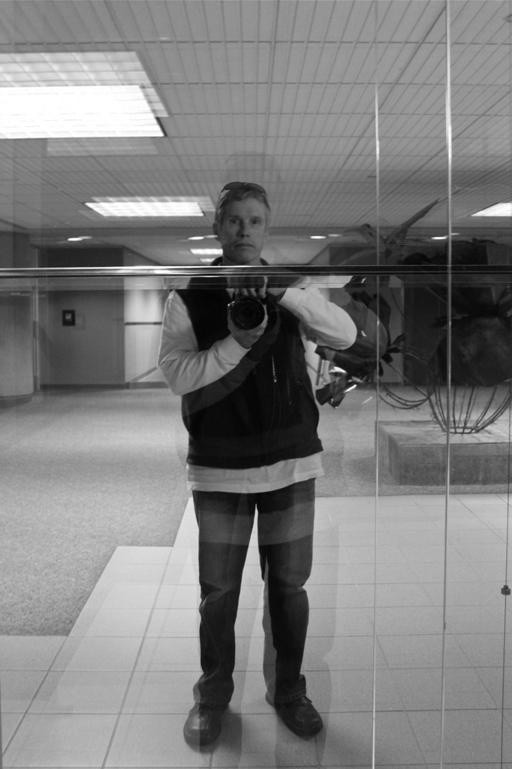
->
[[158, 180, 361, 750]]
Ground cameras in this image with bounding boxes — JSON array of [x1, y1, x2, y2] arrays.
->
[[229, 298, 264, 331]]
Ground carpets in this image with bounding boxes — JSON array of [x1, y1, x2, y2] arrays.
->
[[0, 396, 191, 637]]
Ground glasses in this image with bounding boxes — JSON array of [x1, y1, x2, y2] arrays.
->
[[219, 181, 269, 201]]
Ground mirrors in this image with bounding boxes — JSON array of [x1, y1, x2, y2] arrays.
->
[[0, 0, 512, 769]]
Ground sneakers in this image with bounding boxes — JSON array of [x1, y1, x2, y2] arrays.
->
[[264, 690, 323, 738], [183, 703, 228, 747]]
